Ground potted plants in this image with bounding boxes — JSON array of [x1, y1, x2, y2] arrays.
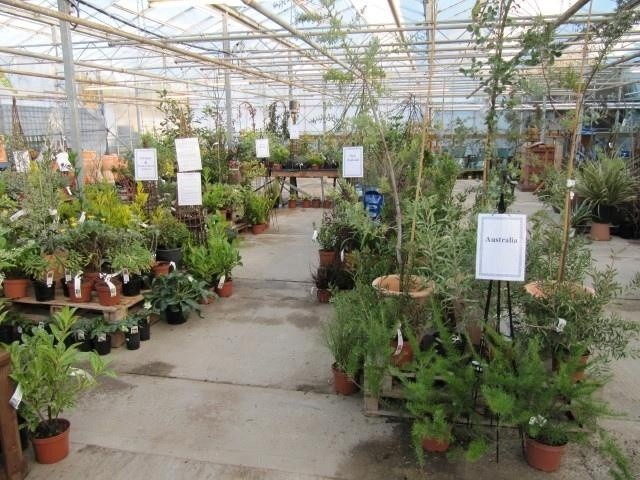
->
[[1, 87, 271, 355], [2, 302, 117, 464], [272, 3, 637, 474]]
